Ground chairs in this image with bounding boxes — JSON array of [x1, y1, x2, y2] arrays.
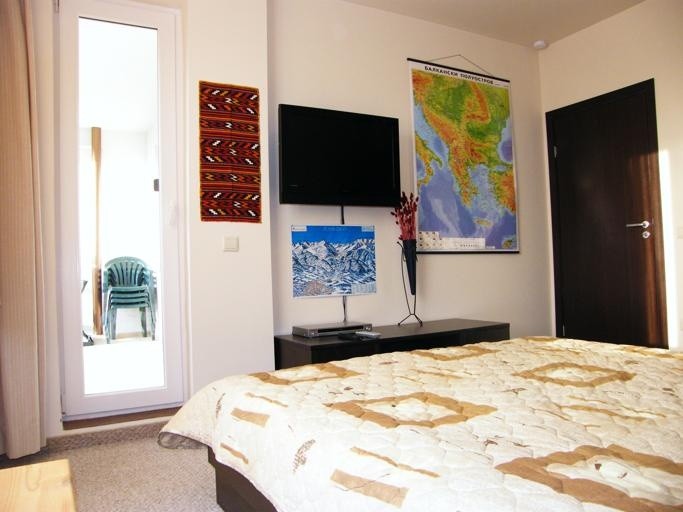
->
[[100, 254, 160, 345]]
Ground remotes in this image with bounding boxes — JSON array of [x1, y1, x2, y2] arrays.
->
[[354, 330, 383, 339]]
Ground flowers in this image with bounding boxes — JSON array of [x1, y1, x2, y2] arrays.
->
[[390, 192, 422, 243]]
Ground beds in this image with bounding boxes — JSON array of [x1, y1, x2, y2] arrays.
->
[[203, 331, 683, 510]]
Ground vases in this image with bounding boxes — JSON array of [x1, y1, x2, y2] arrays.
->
[[400, 240, 425, 295]]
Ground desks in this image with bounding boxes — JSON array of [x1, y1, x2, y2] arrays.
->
[[273, 315, 511, 372]]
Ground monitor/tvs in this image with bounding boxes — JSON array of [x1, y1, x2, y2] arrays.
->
[[278, 102, 401, 210]]
